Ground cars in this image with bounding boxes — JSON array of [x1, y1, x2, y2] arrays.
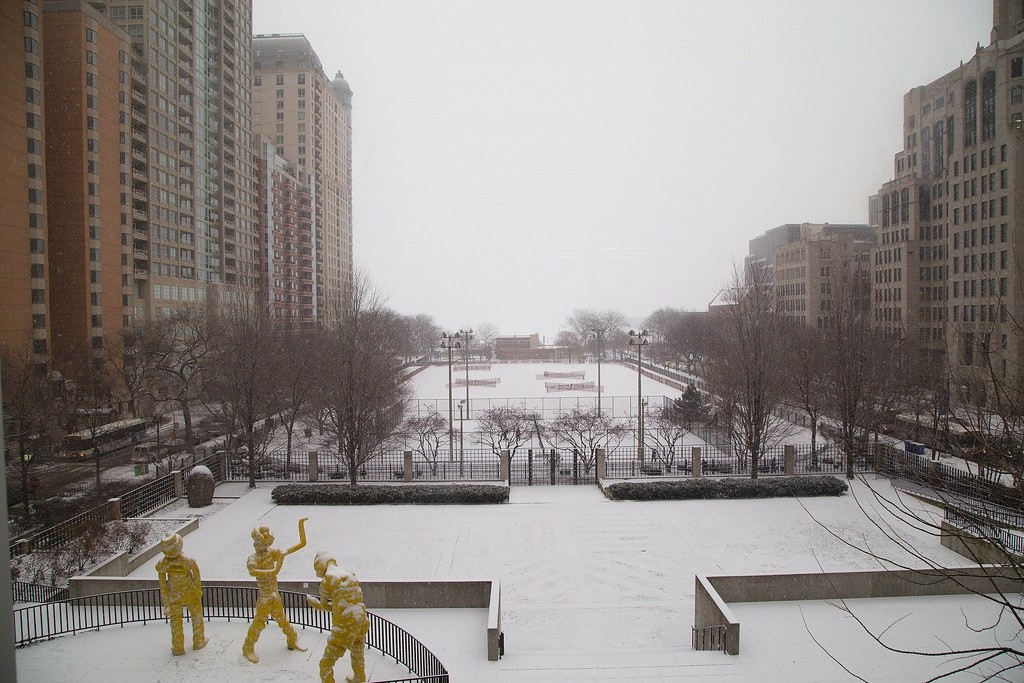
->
[[664, 362, 699, 375], [228, 410, 268, 430], [209, 422, 228, 436], [190, 428, 213, 445], [164, 438, 189, 453]]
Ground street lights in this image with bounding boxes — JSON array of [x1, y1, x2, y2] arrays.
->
[[641, 399, 648, 470], [628, 329, 649, 460], [152, 411, 162, 463], [457, 400, 465, 478], [441, 333, 461, 460], [460, 329, 475, 419], [591, 329, 606, 417]]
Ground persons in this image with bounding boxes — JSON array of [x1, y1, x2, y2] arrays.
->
[[242, 516, 309, 663], [651, 449, 656, 462], [702, 459, 709, 475], [306, 550, 369, 683], [771, 457, 777, 474], [714, 412, 718, 425], [516, 427, 520, 441], [156, 530, 210, 656]]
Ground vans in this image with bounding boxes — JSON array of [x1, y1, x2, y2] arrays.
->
[[131, 441, 170, 464]]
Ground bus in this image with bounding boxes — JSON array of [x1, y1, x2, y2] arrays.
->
[[895, 413, 987, 459], [23, 422, 77, 462], [802, 390, 899, 436], [62, 418, 147, 461]]
